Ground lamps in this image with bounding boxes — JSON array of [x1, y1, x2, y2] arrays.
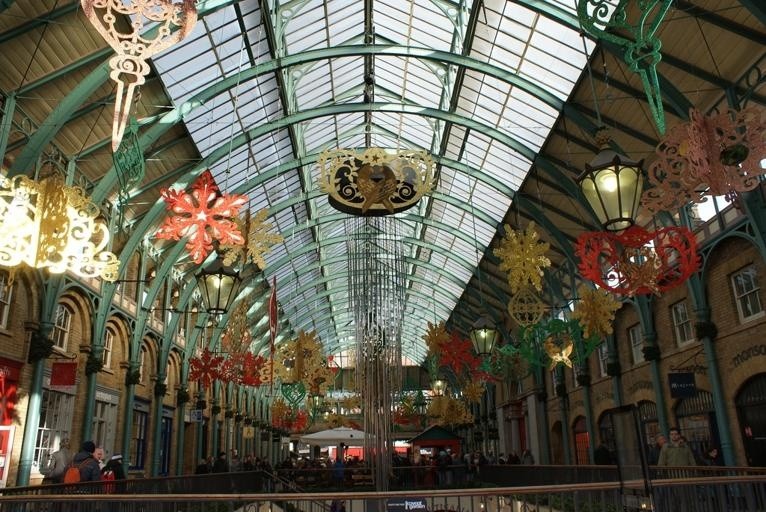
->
[[193, 26, 248, 319], [425, 133, 504, 401], [571, 0, 646, 233]]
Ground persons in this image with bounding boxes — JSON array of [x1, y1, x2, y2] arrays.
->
[[90, 447, 106, 471], [646, 425, 720, 512], [197, 453, 363, 489], [388, 448, 535, 491], [63, 440, 101, 512], [593, 439, 615, 484], [48, 438, 73, 512], [99, 452, 128, 511]]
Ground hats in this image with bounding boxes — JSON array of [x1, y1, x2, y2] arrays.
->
[[110, 453, 123, 461], [82, 440, 95, 454]]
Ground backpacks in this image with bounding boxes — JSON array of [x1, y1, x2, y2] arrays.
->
[[38, 451, 60, 476], [100, 469, 116, 494], [62, 457, 97, 492]]
